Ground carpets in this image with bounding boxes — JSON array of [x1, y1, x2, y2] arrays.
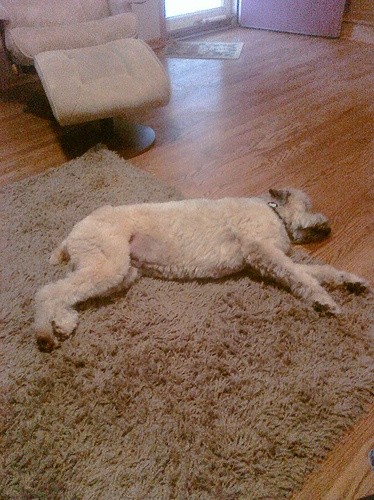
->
[[1, 144, 374, 500]]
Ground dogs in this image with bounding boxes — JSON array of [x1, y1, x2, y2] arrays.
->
[[34, 188, 370, 353]]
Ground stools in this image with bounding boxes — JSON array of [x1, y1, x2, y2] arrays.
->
[[32, 38, 170, 160]]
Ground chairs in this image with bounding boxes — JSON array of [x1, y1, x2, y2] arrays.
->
[[0, 0, 148, 93]]
